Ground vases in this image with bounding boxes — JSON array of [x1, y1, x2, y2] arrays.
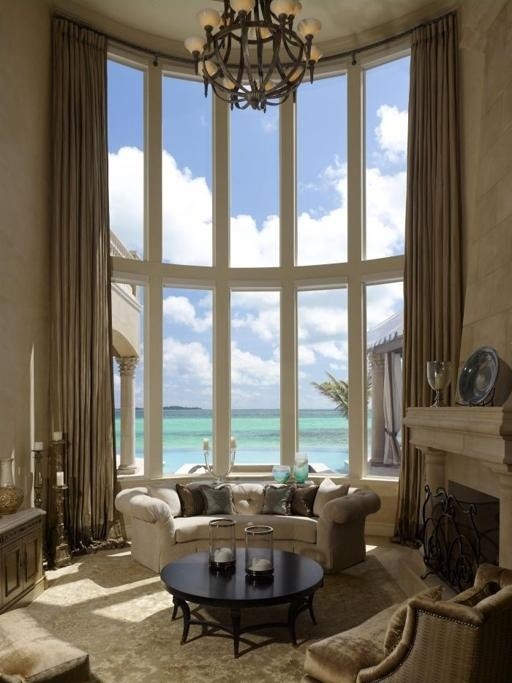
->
[[0, 458, 24, 515]]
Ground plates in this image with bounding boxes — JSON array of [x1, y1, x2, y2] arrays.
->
[[459, 346, 499, 405]]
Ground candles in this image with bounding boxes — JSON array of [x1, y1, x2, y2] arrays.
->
[[57, 472, 63, 486], [203, 438, 208, 450], [35, 442, 42, 449], [230, 437, 236, 448], [54, 432, 62, 441]]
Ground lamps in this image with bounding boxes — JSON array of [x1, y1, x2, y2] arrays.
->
[[184, 0, 323, 113]]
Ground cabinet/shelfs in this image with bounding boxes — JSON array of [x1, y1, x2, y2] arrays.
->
[[1, 512, 47, 614]]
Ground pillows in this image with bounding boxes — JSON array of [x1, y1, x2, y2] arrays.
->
[[151, 488, 182, 517], [292, 485, 318, 516], [384, 584, 442, 656], [313, 478, 350, 517], [199, 485, 233, 515], [450, 581, 501, 606], [176, 484, 205, 517], [262, 485, 295, 515]]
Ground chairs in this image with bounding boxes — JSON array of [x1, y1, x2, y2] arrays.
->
[[303, 563, 512, 683]]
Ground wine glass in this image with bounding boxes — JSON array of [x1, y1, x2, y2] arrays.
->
[[426, 359, 454, 410]]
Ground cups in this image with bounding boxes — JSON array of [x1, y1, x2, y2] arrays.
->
[[245, 525, 274, 577], [273, 465, 291, 484], [294, 451, 309, 484], [208, 519, 237, 568]]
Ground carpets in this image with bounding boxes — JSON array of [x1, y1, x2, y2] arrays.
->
[[0, 547, 408, 683]]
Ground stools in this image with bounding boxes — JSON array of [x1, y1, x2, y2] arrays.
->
[[0, 610, 90, 683]]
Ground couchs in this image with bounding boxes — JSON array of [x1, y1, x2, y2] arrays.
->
[[114, 480, 380, 574]]
